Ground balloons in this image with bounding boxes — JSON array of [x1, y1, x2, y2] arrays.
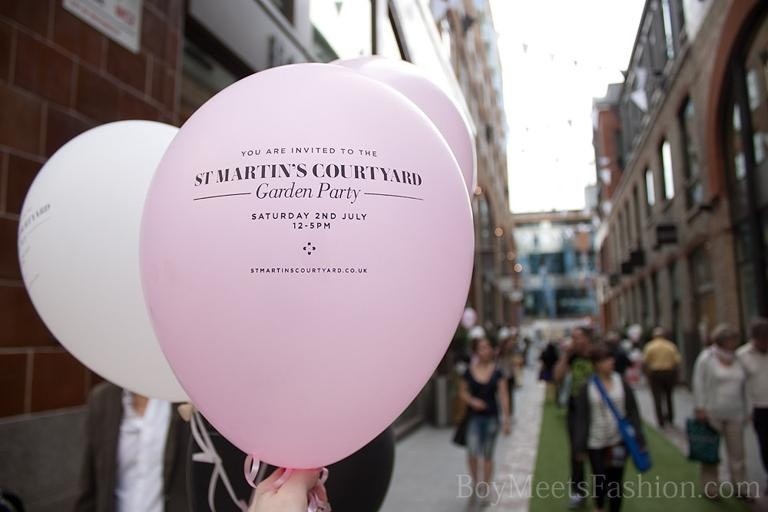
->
[[18, 56, 477, 471]]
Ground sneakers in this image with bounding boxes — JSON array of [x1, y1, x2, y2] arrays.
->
[[568, 493, 586, 510], [468, 488, 490, 508]]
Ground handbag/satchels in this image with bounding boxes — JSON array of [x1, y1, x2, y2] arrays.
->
[[686, 416, 720, 466], [616, 415, 652, 474], [452, 408, 470, 448]]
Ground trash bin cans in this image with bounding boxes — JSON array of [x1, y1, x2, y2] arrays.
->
[[437, 377, 455, 425]]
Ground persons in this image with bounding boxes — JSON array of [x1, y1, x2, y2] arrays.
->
[[71, 380, 394, 512], [454, 323, 768, 512]]
